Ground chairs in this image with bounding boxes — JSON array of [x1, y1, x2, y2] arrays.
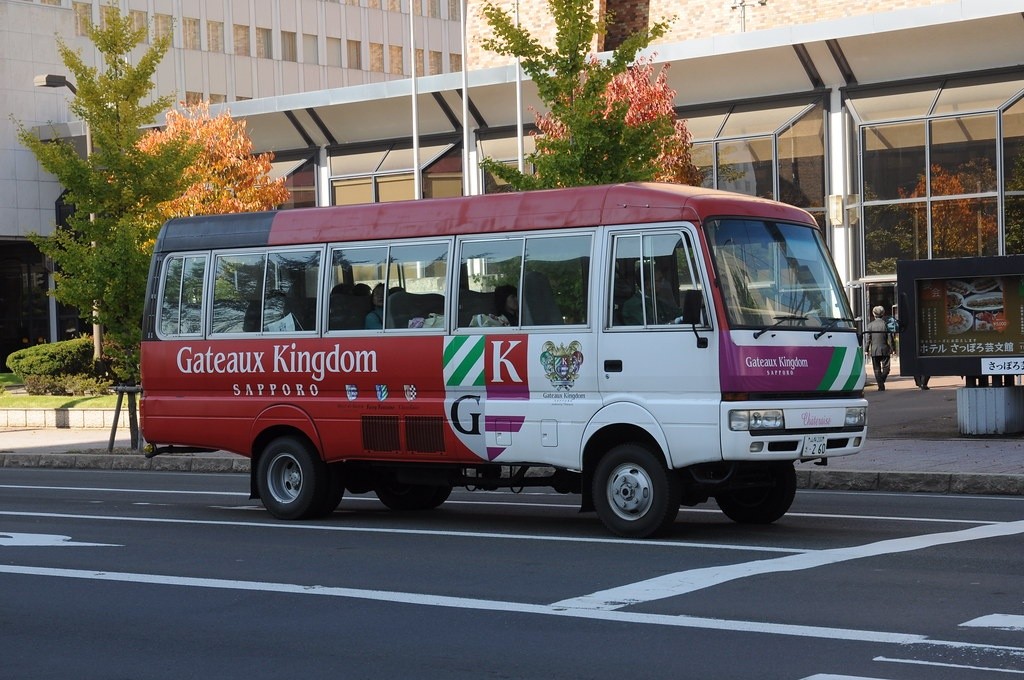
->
[[162, 302, 179, 334], [613, 278, 634, 326], [242, 300, 262, 332], [213, 299, 238, 333], [180, 304, 201, 334], [517, 271, 564, 326]]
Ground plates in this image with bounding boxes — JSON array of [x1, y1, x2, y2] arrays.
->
[[946, 283, 971, 296], [947, 308, 974, 334], [971, 278, 999, 293], [946, 292, 964, 309], [976, 314, 998, 331], [962, 292, 1003, 310]]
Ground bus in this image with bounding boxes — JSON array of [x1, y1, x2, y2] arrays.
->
[[139, 182, 909, 539]]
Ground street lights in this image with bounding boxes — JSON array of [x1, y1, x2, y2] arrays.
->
[[732, 0, 768, 33], [34, 74, 102, 360]]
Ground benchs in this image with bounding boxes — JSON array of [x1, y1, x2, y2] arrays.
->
[[330, 295, 375, 330], [387, 292, 445, 328], [460, 290, 496, 327]]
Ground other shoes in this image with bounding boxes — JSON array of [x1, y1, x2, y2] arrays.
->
[[878, 376, 885, 383], [878, 387, 885, 391], [923, 384, 930, 390], [916, 379, 919, 386]]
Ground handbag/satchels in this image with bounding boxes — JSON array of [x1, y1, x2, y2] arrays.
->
[[408, 312, 444, 328], [469, 313, 511, 327]]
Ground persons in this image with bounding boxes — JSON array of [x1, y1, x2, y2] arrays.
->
[[622, 264, 681, 325], [353, 284, 372, 295], [864, 306, 896, 391], [495, 285, 518, 326], [365, 283, 384, 329], [914, 376, 930, 390]]
